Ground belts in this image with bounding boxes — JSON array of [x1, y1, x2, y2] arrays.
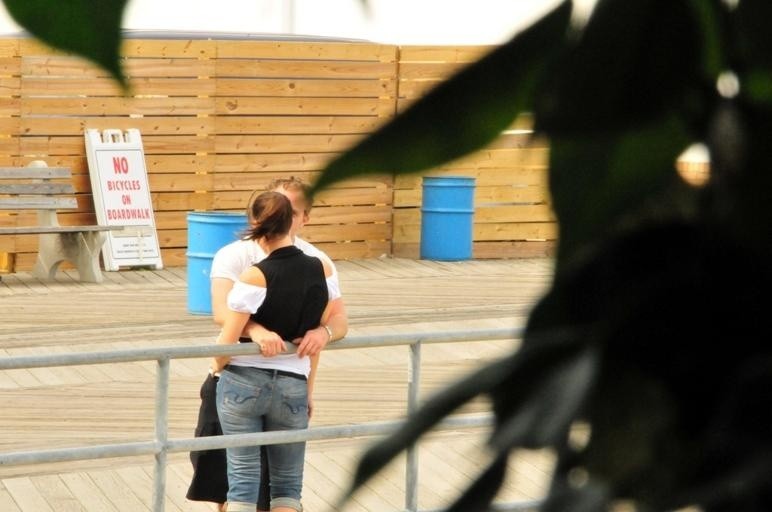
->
[[249, 367, 308, 382]]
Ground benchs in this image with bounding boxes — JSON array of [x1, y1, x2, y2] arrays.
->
[[0, 161, 122, 284]]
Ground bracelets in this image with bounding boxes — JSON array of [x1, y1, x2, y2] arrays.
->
[[323, 325, 333, 342]]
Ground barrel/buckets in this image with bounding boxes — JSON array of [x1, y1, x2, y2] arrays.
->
[[420, 175, 477, 261], [185, 210, 251, 315]]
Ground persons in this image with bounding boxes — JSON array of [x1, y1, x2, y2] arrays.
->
[[186, 180, 348, 511], [211, 192, 335, 512]]
